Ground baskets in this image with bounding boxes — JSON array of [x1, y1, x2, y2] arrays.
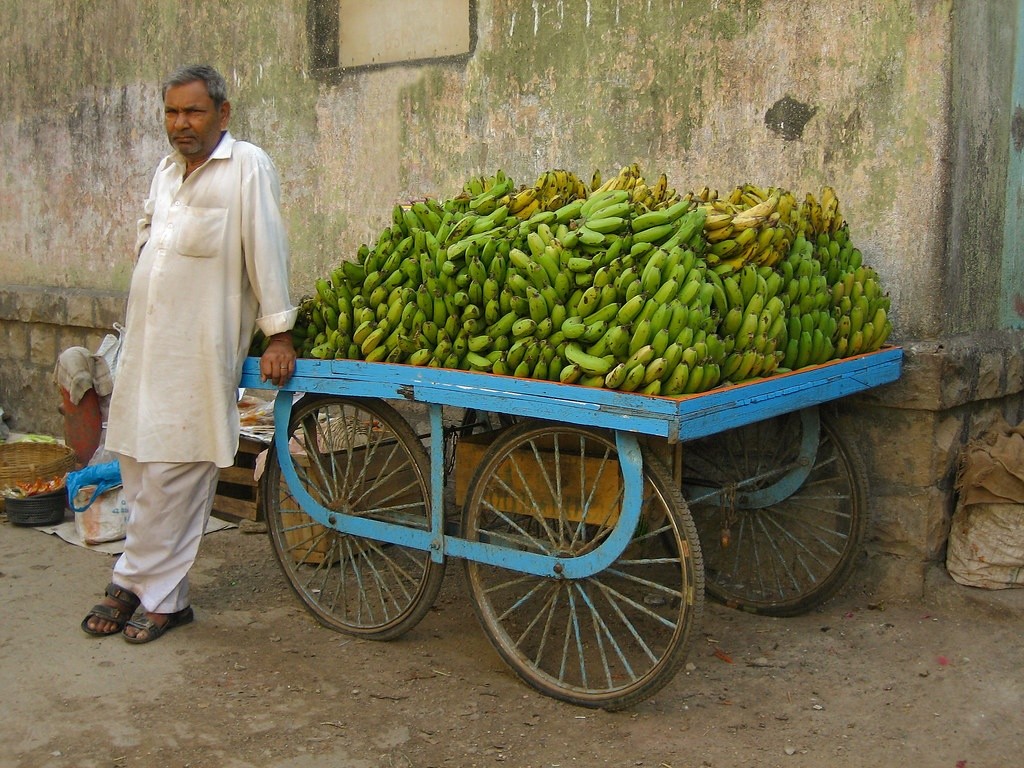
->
[[5, 484, 68, 525], [0, 440, 77, 493]]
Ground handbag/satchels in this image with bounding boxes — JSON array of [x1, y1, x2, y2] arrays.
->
[[73, 483, 131, 544], [66, 460, 122, 512]]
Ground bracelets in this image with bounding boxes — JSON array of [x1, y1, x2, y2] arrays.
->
[[273, 336, 293, 345]]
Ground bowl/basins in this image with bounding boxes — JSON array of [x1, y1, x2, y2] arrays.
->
[[5, 488, 66, 524]]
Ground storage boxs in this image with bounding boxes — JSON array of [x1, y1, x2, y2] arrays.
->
[[211, 434, 267, 523], [279, 432, 425, 564], [455, 423, 621, 528]]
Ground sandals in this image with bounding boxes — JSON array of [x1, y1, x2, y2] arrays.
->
[[121, 605, 194, 644], [81, 582, 140, 637]]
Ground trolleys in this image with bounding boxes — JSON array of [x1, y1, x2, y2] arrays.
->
[[231, 347, 906, 715]]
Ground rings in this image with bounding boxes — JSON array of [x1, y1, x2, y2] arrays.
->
[[281, 367, 287, 369]]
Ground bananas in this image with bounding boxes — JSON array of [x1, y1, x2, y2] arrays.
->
[[247, 164, 897, 398]]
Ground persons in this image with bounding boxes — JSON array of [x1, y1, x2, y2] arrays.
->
[[81, 65, 298, 644]]
